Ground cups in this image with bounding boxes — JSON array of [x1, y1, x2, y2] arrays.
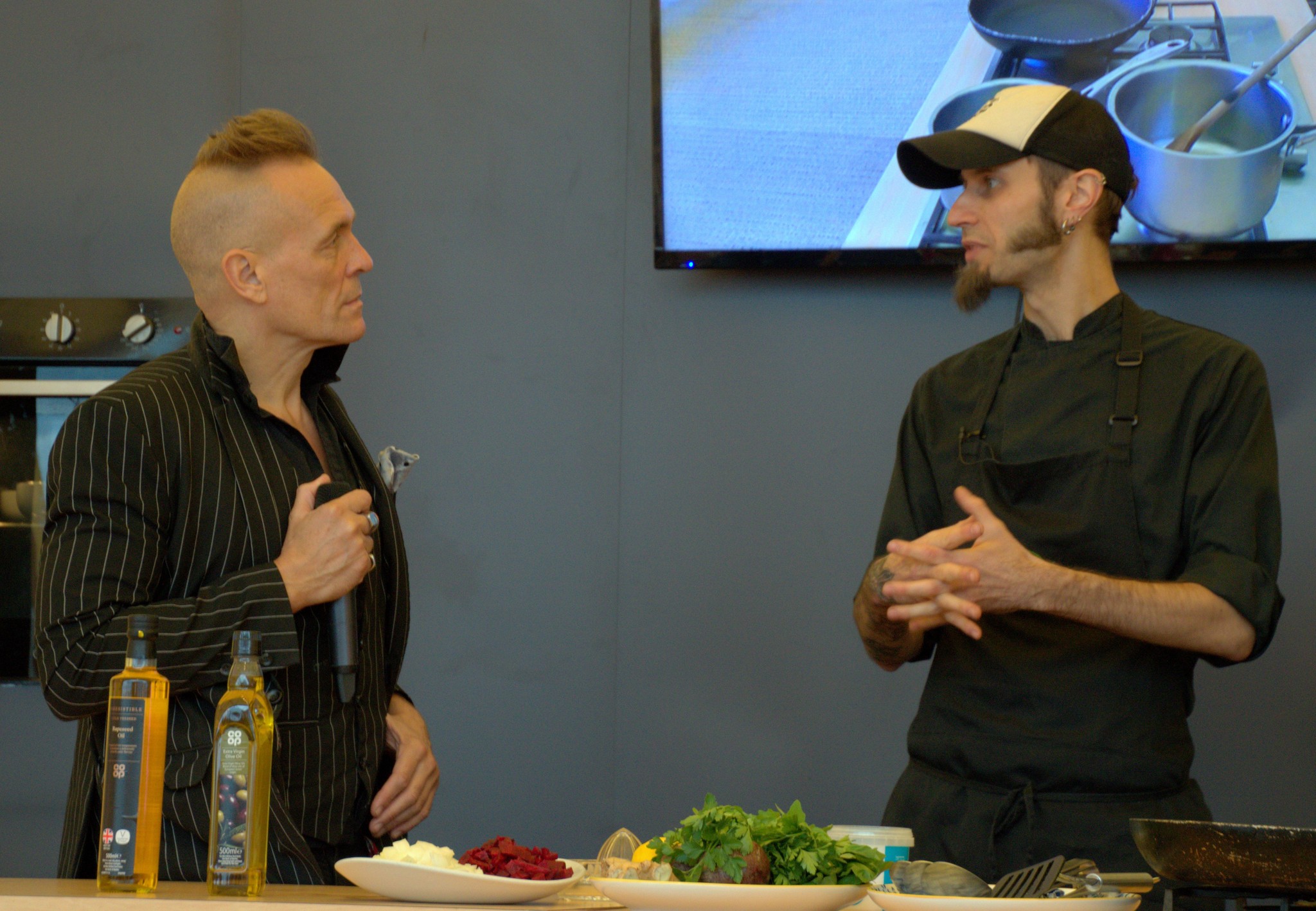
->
[[826, 824, 914, 911]]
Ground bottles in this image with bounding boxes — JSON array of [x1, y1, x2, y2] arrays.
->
[[98, 613, 169, 898], [207, 630, 274, 897]]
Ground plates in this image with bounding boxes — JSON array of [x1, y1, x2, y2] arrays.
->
[[1127, 816, 1315, 892], [867, 885, 1138, 911], [332, 855, 584, 904], [589, 874, 873, 911]]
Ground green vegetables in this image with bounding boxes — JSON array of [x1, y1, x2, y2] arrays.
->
[[648, 793, 886, 884]]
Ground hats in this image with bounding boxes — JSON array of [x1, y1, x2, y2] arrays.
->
[[895, 82, 1132, 208]]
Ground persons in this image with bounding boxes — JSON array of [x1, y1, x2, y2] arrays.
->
[[29, 111, 440, 888], [849, 84, 1287, 886]]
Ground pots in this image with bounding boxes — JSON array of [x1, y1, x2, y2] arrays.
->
[[926, 0, 1299, 236]]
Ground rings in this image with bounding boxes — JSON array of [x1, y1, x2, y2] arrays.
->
[[368, 553, 375, 571], [359, 510, 378, 535]]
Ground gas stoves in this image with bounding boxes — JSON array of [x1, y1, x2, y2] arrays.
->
[[908, 9, 1313, 243]]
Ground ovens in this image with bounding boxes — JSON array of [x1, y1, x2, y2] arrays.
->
[[0, 290, 202, 683]]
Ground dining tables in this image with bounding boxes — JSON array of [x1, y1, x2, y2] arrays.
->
[[0, 878, 884, 911]]
